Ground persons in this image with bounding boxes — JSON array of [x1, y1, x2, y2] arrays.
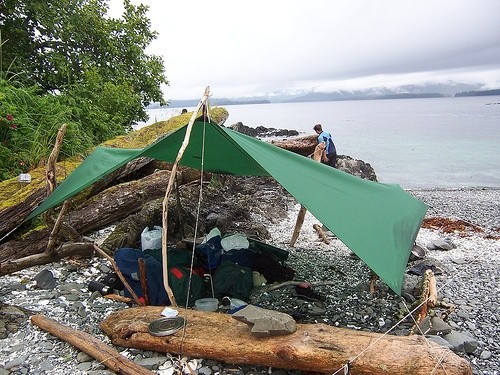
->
[[181, 108, 187, 114], [314, 124, 336, 168]]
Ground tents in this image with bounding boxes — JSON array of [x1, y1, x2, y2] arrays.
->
[[0, 86, 500, 375]]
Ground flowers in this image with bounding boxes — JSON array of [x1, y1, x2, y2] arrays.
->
[[0, 114, 17, 129]]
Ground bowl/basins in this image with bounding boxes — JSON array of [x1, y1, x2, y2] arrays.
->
[[195, 298, 220, 312]]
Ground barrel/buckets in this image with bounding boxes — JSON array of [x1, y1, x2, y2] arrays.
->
[[409, 244, 426, 263]]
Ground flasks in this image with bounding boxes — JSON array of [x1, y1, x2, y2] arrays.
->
[[87, 281, 112, 295]]
